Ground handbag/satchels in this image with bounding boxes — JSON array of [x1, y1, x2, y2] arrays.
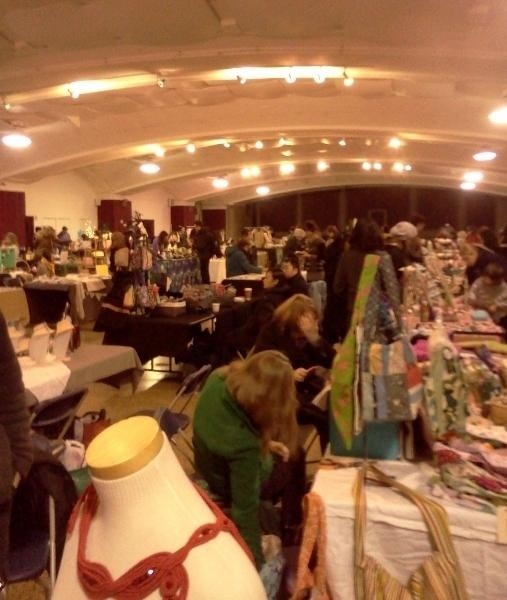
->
[[325, 249, 426, 463]]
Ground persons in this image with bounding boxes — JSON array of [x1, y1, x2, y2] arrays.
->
[[43, 413, 269, 600], [0, 311, 32, 582], [192, 347, 305, 600], [0, 209, 507, 456]]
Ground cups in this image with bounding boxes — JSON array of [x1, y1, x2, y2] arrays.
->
[[244, 287, 253, 302], [212, 302, 220, 313]]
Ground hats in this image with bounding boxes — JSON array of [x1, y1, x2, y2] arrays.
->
[[390, 220, 419, 241], [350, 217, 378, 240]]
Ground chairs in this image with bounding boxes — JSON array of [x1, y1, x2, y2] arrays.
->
[[0, 461, 66, 599], [192, 438, 232, 509]]
